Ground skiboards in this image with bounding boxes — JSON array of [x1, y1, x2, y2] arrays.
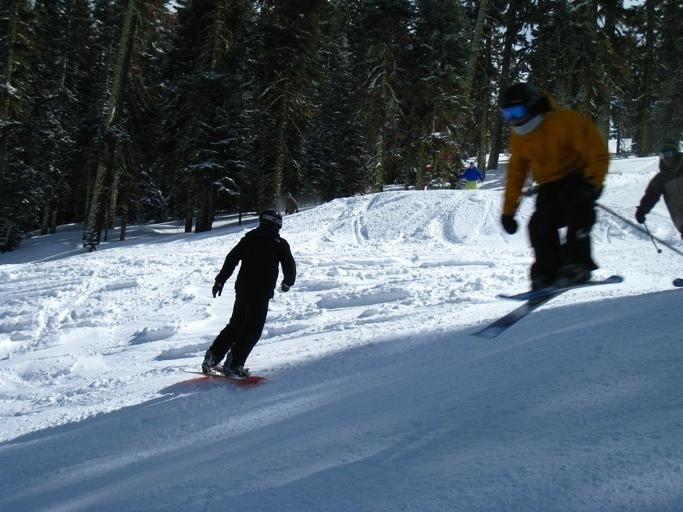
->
[[470, 275, 624, 339]]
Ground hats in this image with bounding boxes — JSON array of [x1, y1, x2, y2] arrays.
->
[[497, 83, 537, 109], [259, 209, 282, 229]]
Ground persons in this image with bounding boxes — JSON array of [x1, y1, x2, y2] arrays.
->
[[497, 81, 609, 290], [200, 208, 296, 378], [456, 160, 483, 189], [634, 136, 683, 242]]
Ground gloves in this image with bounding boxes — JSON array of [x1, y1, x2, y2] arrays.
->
[[635, 207, 646, 223], [501, 214, 518, 234], [276, 279, 291, 293], [577, 188, 595, 206], [212, 280, 223, 298]]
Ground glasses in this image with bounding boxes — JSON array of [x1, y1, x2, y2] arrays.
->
[[659, 150, 673, 160], [498, 100, 530, 121]]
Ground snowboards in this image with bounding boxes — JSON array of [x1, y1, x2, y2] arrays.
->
[[186, 368, 264, 389]]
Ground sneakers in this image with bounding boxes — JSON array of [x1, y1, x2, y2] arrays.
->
[[202, 348, 250, 377], [531, 257, 592, 296]]
